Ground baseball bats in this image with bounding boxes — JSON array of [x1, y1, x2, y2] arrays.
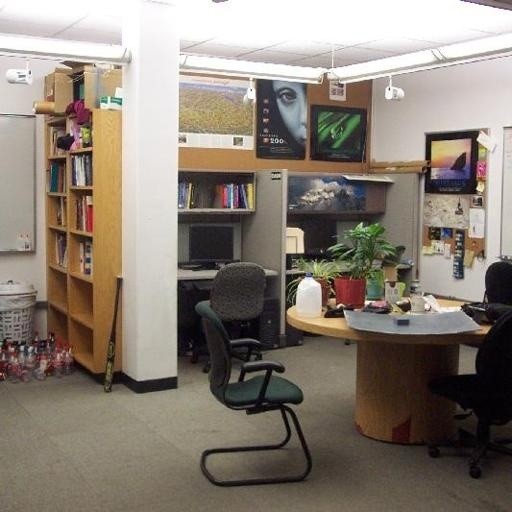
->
[[103, 275, 123, 393]]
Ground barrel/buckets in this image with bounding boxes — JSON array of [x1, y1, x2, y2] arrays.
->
[[297, 273, 323, 318]]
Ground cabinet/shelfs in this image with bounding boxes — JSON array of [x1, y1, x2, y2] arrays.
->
[[177, 171, 286, 347], [44, 109, 122, 373], [464, 262, 512, 306], [287, 171, 416, 286]]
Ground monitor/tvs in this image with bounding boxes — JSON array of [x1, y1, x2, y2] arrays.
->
[[189, 226, 234, 271]]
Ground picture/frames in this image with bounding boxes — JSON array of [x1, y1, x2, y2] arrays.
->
[[310, 104, 367, 162], [425, 131, 478, 194]]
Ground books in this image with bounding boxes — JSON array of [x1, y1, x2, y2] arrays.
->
[[47, 125, 94, 277], [179, 178, 255, 211]]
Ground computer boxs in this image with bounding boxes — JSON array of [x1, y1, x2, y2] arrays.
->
[[241, 298, 281, 350]]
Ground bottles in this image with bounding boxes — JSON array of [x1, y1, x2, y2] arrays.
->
[[408, 278, 424, 296], [0, 331, 76, 382], [384, 278, 399, 304]]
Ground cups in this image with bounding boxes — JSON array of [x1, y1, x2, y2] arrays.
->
[[410, 296, 431, 312]]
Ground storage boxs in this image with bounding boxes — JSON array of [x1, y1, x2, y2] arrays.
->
[[44, 66, 121, 113]]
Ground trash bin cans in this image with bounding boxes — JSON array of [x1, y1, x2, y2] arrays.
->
[[0, 286, 38, 343]]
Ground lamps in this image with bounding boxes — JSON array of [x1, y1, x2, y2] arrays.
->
[[384, 75, 405, 100], [6, 59, 33, 85], [246, 78, 256, 100]]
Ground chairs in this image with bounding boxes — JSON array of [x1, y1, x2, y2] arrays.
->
[[427, 310, 512, 479], [191, 262, 312, 486]]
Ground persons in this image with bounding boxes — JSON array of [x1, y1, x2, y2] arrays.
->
[[271, 80, 307, 149]]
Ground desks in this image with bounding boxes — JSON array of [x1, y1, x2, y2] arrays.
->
[[286, 297, 493, 445]]
